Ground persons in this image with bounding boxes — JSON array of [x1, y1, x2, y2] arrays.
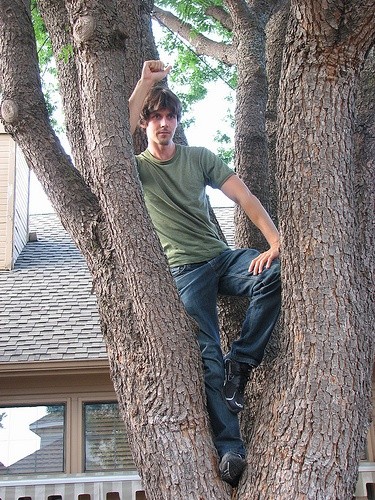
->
[[127, 60, 281, 487]]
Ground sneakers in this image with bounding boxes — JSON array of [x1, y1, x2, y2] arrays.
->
[[216, 451, 246, 489], [222, 350, 251, 414]]
